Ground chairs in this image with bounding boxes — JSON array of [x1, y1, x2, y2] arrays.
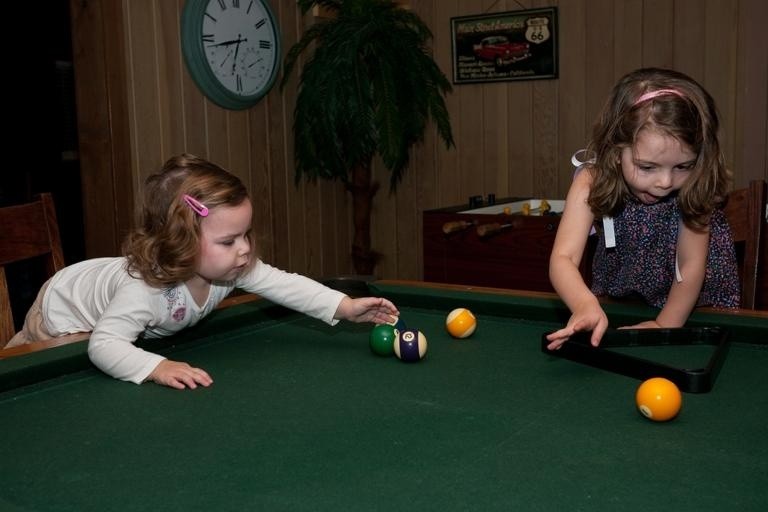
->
[[1, 193, 67, 344], [719, 180, 767, 308]]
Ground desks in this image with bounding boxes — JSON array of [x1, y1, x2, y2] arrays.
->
[[0, 273, 767, 511]]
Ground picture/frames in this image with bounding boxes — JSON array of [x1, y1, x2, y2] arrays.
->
[[449, 6, 558, 84]]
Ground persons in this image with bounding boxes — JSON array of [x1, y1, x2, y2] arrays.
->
[[5, 154, 401, 390], [546, 67, 741, 352]]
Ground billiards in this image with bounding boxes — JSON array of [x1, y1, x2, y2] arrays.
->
[[370, 314, 428, 362], [447, 308, 476, 339], [635, 378, 682, 421]]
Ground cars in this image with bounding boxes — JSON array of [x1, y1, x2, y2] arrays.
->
[[472, 35, 532, 67]]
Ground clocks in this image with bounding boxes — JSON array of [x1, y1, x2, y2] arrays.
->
[[179, 1, 281, 111]]
